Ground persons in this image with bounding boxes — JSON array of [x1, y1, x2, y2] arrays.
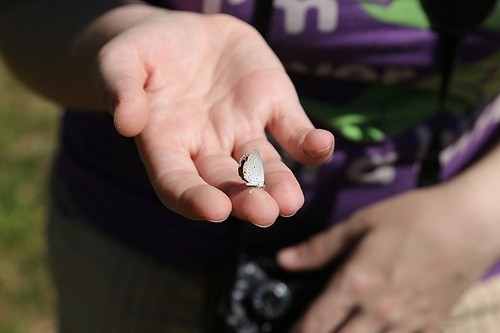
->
[[1, 0, 499, 333]]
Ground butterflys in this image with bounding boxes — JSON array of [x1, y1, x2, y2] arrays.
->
[[238, 149, 266, 194]]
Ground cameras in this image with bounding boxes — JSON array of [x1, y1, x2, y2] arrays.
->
[[207, 246, 332, 332]]
